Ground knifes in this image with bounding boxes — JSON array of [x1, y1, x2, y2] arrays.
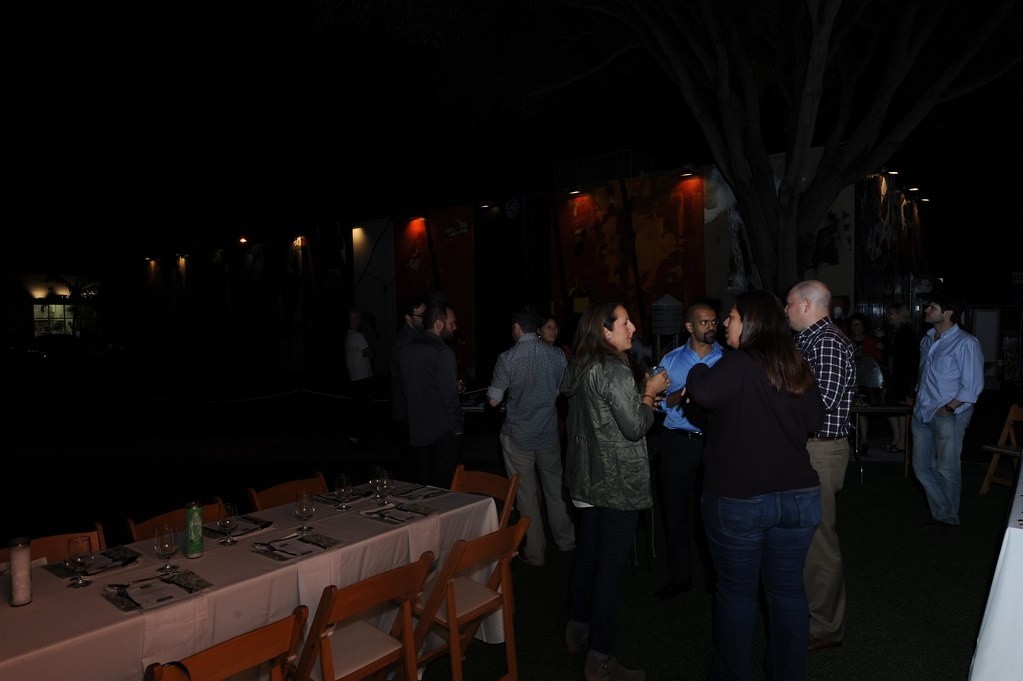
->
[[161, 577, 195, 593]]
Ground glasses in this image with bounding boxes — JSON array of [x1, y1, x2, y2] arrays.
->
[[411, 312, 424, 319]]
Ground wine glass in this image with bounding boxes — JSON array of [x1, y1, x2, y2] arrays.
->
[[153, 520, 179, 575], [295, 499, 316, 534], [333, 476, 352, 512], [216, 502, 239, 545], [65, 535, 94, 588]]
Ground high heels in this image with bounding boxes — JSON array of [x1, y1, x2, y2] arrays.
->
[[857, 444, 869, 458]]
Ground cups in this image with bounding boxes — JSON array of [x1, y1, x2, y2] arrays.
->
[[649, 365, 667, 402], [455, 376, 465, 394], [378, 479, 397, 505]]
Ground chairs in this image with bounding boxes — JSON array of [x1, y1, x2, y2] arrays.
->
[[981, 404, 1023, 494], [0, 462, 531, 681]]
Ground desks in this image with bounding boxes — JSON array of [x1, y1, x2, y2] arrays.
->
[[848, 401, 913, 486], [0, 480, 505, 681]]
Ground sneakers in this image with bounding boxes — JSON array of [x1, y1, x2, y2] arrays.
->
[[567, 643, 584, 653], [585, 654, 646, 681]]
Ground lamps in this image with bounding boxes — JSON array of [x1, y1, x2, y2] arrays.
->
[[480, 202, 489, 208], [679, 169, 693, 177], [881, 167, 903, 175], [900, 183, 921, 191], [914, 193, 929, 202], [569, 187, 580, 194]]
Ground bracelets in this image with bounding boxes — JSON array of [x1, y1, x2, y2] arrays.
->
[[945, 404, 955, 412], [642, 394, 654, 399]]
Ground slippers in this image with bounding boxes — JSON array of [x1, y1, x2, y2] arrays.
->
[[880, 443, 905, 453]]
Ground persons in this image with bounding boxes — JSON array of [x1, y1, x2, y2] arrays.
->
[[687, 290, 825, 681], [567, 301, 671, 681], [785, 280, 856, 651], [346, 309, 375, 444], [390, 301, 464, 487], [489, 305, 574, 566], [831, 302, 921, 453], [654, 305, 732, 598], [912, 298, 984, 533]]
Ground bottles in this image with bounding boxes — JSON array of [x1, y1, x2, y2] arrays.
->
[[874, 319, 883, 338]]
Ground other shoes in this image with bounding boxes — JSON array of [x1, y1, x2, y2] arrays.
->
[[653, 580, 691, 603], [810, 632, 842, 652], [516, 549, 528, 563], [913, 519, 960, 537]]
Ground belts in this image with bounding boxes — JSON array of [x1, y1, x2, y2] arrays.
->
[[675, 428, 704, 440]]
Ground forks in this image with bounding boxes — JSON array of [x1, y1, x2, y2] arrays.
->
[[268, 543, 297, 556], [117, 588, 140, 607]]
